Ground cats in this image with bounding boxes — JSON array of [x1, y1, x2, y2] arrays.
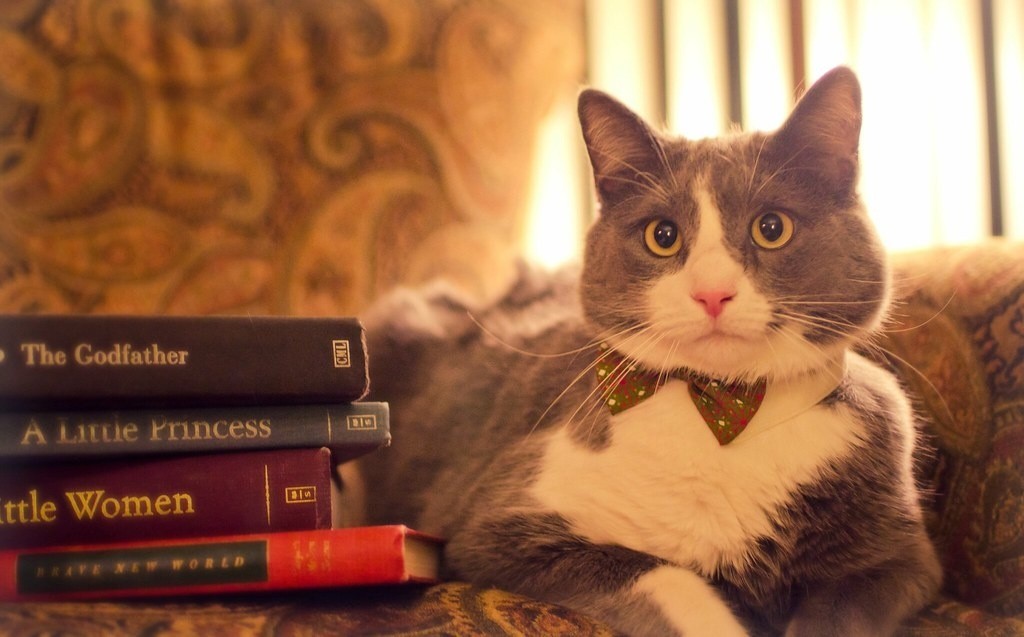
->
[[334, 66, 936, 637]]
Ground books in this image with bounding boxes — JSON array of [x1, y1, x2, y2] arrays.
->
[[0, 446, 331, 550], [0, 523, 451, 600], [0, 314, 371, 402], [0, 402, 391, 466]]
